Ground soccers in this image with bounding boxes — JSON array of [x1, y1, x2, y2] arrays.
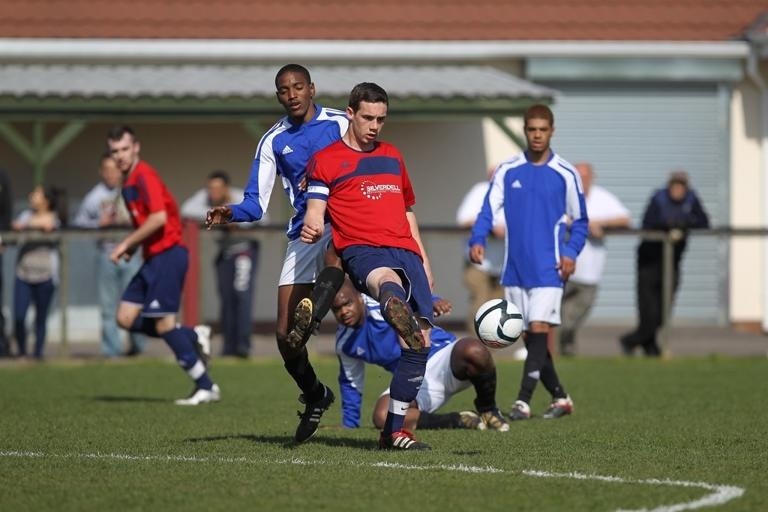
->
[[474, 299, 524, 349]]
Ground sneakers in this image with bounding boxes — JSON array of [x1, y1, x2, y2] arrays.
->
[[379, 429, 432, 452], [286, 297, 314, 351], [458, 391, 574, 433], [173, 383, 220, 406], [295, 384, 333, 444], [384, 297, 424, 353], [193, 325, 212, 361]]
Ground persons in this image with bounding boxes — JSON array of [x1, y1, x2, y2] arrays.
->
[[617, 170, 713, 356], [332, 276, 512, 434], [456, 166, 508, 319], [300, 83, 435, 450], [468, 105, 589, 419], [74, 156, 149, 358], [12, 181, 61, 363], [179, 170, 267, 363], [555, 164, 638, 358], [202, 65, 353, 441], [105, 124, 221, 407]]
